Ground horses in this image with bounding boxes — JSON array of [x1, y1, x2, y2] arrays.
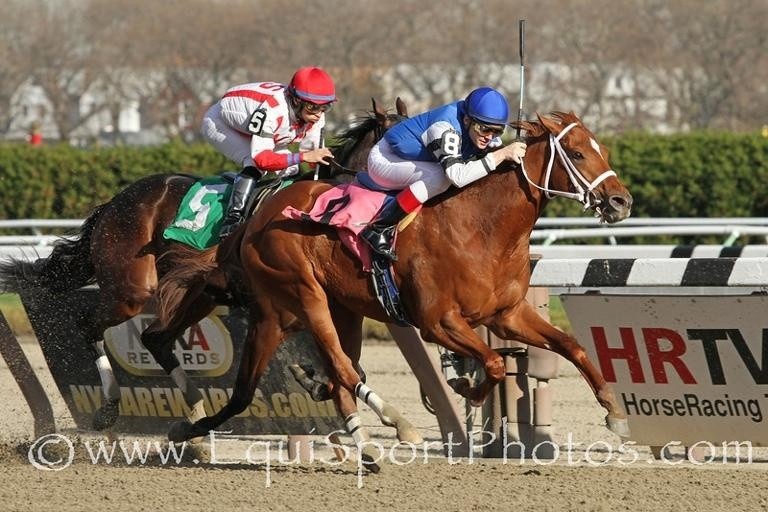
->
[[0, 97, 420, 444], [132, 110, 632, 476]]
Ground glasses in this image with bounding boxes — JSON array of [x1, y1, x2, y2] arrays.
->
[[471, 121, 504, 136], [299, 101, 332, 114]]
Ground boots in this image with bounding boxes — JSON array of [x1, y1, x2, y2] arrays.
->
[[359, 185, 423, 261], [218, 173, 256, 238]]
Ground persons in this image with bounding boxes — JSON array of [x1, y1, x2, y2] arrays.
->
[[358, 88, 527, 258], [203, 67, 338, 236]]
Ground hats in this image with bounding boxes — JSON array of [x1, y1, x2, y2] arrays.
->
[[288, 67, 338, 104], [464, 86, 509, 124]]
